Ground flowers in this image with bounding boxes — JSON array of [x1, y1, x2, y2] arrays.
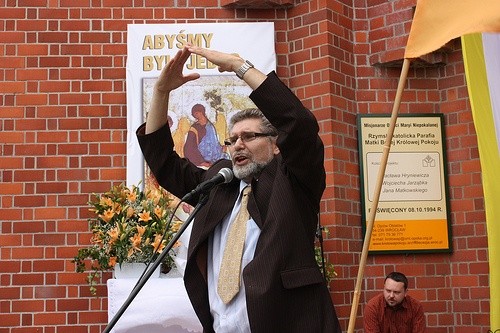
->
[[71, 176, 183, 297]]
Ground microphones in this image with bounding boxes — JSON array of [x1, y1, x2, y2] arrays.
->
[[182, 167, 234, 200]]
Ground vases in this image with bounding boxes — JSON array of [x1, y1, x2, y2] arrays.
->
[[113, 263, 162, 278]]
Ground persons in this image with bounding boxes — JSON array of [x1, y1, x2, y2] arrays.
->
[[363, 272, 426, 333], [134, 44, 343, 333]]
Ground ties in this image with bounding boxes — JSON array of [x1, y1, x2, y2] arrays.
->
[[217, 184, 252, 305]]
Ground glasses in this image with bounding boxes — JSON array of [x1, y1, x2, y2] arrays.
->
[[224, 132, 278, 147]]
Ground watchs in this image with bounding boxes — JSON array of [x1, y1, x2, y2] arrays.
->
[[235, 59, 254, 79]]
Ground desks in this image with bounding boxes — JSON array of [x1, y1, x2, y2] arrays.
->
[[106, 277, 203, 333]]
